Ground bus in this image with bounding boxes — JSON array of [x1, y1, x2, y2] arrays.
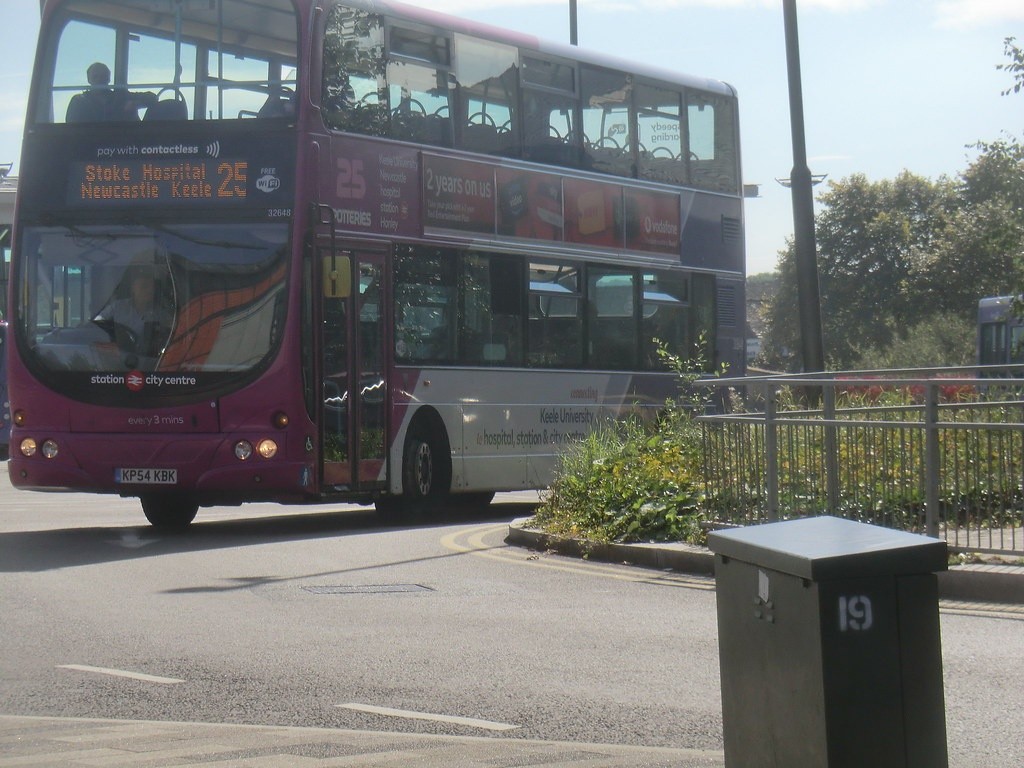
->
[[975, 293, 1024, 404], [0, 0, 745, 526]]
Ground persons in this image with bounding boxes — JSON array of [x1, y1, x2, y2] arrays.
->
[[92, 272, 162, 356], [65, 62, 158, 123]]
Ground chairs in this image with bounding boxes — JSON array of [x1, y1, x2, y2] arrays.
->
[[143, 86, 188, 123], [239, 87, 298, 120], [333, 89, 734, 192]]
